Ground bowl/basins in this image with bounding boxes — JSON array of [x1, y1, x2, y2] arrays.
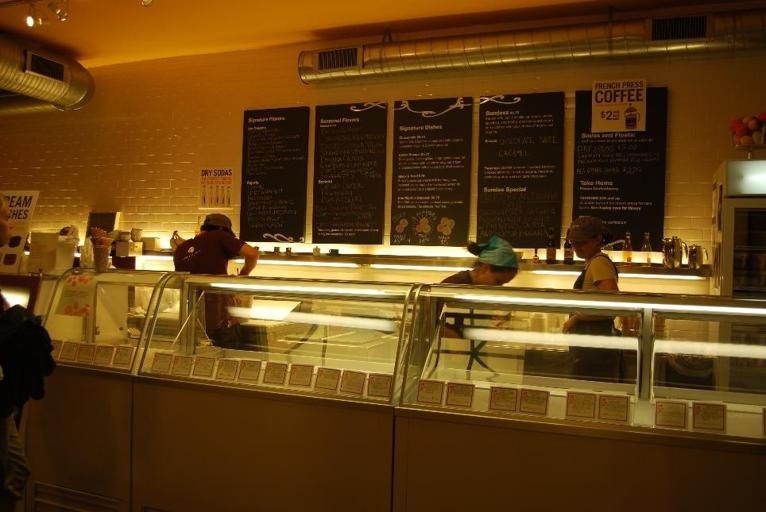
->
[[120, 231, 131, 242]]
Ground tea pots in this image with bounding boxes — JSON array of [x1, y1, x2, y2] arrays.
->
[[684, 242, 708, 271], [661, 237, 687, 270]]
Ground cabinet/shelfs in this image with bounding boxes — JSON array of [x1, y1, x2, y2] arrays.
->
[[0, 273, 61, 435], [129, 273, 427, 511], [23, 267, 191, 512], [392, 283, 766, 511]]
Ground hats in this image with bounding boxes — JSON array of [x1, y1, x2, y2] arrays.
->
[[204, 214, 238, 236], [567, 217, 603, 241]]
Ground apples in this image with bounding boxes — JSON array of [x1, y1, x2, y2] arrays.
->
[[731, 111, 765, 145]]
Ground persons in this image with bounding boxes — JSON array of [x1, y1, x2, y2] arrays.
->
[[0, 293, 31, 511], [563, 214, 619, 379], [174, 213, 259, 351], [426, 233, 518, 338]]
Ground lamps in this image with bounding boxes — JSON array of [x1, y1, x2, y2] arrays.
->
[[52, 2, 68, 22], [26, 4, 49, 27], [141, 0, 152, 6]]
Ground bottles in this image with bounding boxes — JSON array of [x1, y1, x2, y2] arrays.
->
[[643, 231, 654, 266], [564, 226, 576, 265], [546, 228, 558, 265], [623, 232, 634, 265]]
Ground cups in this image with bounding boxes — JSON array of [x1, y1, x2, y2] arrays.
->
[[329, 248, 339, 255]]
